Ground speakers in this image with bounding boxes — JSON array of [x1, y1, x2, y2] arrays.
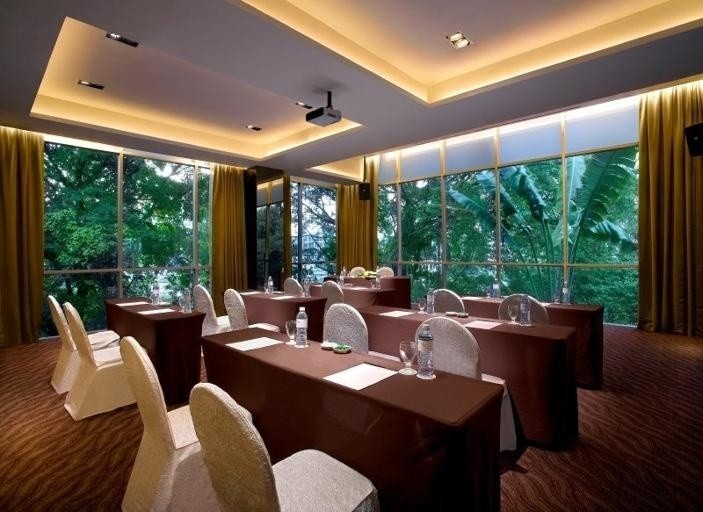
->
[[684, 123, 703, 157], [358, 183, 370, 200]]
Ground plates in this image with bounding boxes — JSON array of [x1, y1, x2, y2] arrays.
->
[[320, 342, 351, 353], [446, 311, 469, 317]]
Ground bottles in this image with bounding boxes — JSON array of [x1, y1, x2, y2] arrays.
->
[[268, 276, 273, 295], [427, 287, 434, 315], [561, 281, 570, 304], [152, 280, 159, 306], [493, 277, 501, 299], [183, 287, 192, 313], [520, 293, 531, 324], [295, 307, 308, 346], [418, 323, 433, 377]]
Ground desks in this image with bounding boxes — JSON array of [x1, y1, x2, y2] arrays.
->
[[238, 290, 328, 340], [313, 274, 413, 309], [103, 295, 207, 406], [458, 293, 604, 392], [198, 321, 505, 511], [349, 302, 582, 450]]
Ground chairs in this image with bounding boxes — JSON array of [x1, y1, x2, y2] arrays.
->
[[376, 267, 394, 277], [223, 288, 280, 334], [62, 300, 146, 420], [193, 284, 231, 356], [320, 281, 345, 312], [426, 289, 466, 316], [413, 316, 526, 474], [349, 267, 366, 276], [322, 304, 369, 354], [284, 278, 303, 296], [497, 293, 550, 327], [117, 335, 253, 512], [46, 294, 122, 396], [188, 382, 381, 512]]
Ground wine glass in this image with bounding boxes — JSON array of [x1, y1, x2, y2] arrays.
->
[[399, 341, 417, 376], [417, 297, 426, 314], [286, 320, 296, 345], [508, 304, 520, 323]]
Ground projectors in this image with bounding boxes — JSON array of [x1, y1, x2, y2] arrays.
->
[[305, 107, 342, 128]]
